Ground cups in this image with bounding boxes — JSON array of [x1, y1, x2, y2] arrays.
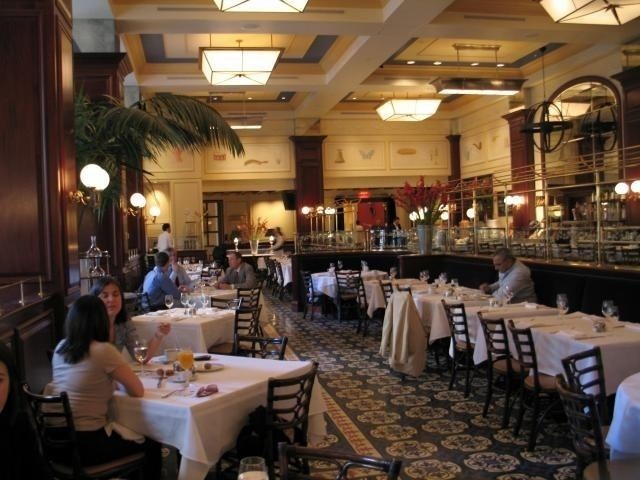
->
[[165, 348, 179, 359], [238, 456, 269, 479]]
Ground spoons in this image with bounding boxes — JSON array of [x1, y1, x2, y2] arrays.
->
[[162, 383, 189, 398]]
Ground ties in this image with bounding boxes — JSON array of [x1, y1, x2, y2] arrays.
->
[[176, 276, 179, 287]]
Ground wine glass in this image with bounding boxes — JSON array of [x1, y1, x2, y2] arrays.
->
[[135, 339, 151, 377], [164, 292, 209, 316], [327, 260, 514, 312], [600, 299, 620, 327], [177, 350, 194, 397], [555, 294, 569, 323], [179, 256, 217, 292]]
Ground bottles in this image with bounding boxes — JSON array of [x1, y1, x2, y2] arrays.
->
[[128, 249, 140, 269], [87, 235, 102, 256], [590, 192, 628, 220], [173, 363, 187, 382], [92, 258, 106, 275]]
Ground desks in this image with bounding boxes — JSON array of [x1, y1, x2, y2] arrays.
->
[[128, 306, 254, 356], [410, 284, 627, 403], [178, 286, 238, 311], [107, 351, 326, 478], [309, 268, 387, 303], [359, 277, 429, 318], [173, 262, 227, 280]]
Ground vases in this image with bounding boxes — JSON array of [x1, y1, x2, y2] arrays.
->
[[415, 224, 434, 255], [248, 238, 260, 255]]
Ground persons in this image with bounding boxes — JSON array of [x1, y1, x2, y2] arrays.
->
[[479, 248, 538, 304], [0, 342, 68, 480], [388, 217, 405, 246], [40, 294, 163, 480], [141, 223, 195, 313], [212, 252, 256, 291], [88, 275, 171, 365], [267, 227, 285, 255]]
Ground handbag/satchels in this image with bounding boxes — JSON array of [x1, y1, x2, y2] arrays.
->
[[235, 406, 303, 459]]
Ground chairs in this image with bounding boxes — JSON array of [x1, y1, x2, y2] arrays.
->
[[507, 319, 568, 454], [299, 256, 441, 386], [21, 383, 148, 478], [555, 372, 639, 478], [132, 255, 289, 362], [277, 441, 402, 479], [476, 312, 530, 428], [442, 299, 489, 399], [216, 361, 318, 479], [560, 345, 630, 479]]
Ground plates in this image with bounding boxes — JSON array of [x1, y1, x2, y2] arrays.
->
[[194, 355, 211, 362], [196, 363, 223, 371]]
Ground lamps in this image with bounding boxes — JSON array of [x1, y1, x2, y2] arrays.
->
[[548, 99, 589, 117], [429, 76, 529, 96], [69, 164, 110, 207], [615, 182, 640, 204], [372, 94, 448, 123], [197, 46, 287, 85], [126, 193, 146, 218], [210, 117, 263, 130], [211, 0, 309, 13], [539, 0, 640, 28]]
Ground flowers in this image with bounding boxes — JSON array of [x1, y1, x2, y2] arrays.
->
[[236, 215, 269, 238], [394, 176, 449, 223]]
